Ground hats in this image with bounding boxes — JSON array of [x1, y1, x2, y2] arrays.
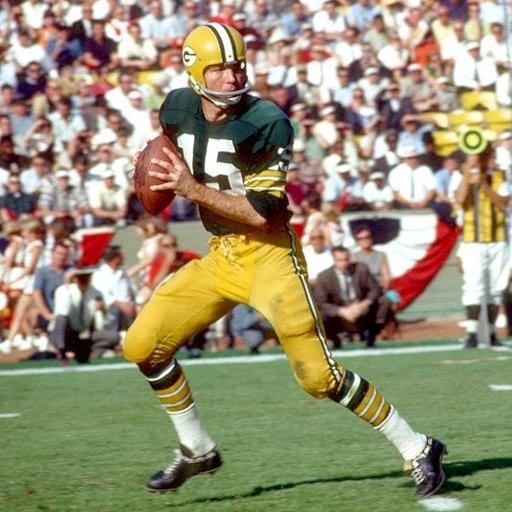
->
[[268, 24, 397, 183], [56, 170, 71, 180], [397, 31, 477, 160], [101, 169, 115, 180]]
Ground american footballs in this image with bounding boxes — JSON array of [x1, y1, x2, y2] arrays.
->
[[134, 135, 180, 215]]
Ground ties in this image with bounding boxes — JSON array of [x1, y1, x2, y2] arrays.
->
[[342, 275, 351, 302], [79, 292, 88, 330]]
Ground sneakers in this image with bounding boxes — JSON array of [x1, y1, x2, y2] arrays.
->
[[146, 443, 223, 493], [489, 332, 501, 345], [1, 330, 50, 354], [466, 333, 478, 346], [404, 434, 448, 501]]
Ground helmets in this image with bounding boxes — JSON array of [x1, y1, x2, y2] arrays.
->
[[181, 22, 252, 108]]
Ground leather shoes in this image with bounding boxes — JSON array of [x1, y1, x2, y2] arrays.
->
[[331, 335, 376, 350]]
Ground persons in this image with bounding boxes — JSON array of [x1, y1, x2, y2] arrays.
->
[[120, 21, 449, 501], [1, 1, 511, 365]]
